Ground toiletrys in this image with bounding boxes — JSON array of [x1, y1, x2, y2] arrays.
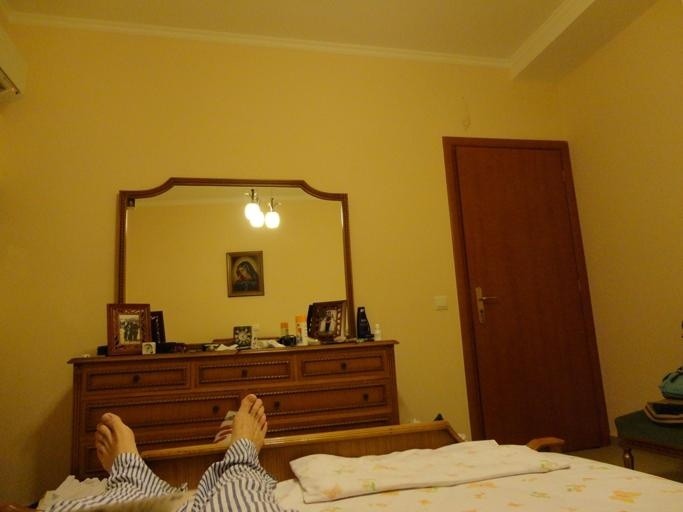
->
[[296, 315, 309, 346], [373, 323, 382, 342], [281, 321, 288, 337]]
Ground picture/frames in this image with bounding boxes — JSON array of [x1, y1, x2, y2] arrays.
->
[[225, 250, 264, 296], [311, 299, 347, 343], [306, 304, 312, 336], [106, 303, 152, 356], [149, 311, 164, 352]]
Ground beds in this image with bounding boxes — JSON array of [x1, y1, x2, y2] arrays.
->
[[37, 421, 682, 512]]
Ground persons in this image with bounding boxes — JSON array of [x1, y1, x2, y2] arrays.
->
[[324, 310, 332, 332], [232, 261, 258, 293], [0, 394, 298, 512], [120, 318, 138, 342]]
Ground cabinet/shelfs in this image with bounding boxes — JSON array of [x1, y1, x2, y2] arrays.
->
[[65, 338, 401, 485]]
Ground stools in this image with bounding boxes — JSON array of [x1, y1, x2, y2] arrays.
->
[[615, 404, 683, 477]]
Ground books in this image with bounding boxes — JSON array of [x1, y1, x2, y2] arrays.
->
[[643, 397, 683, 424]]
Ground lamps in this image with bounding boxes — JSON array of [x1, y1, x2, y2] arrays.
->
[[242, 186, 280, 231]]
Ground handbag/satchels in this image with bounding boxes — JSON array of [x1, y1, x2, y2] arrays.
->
[[660, 366, 683, 398]]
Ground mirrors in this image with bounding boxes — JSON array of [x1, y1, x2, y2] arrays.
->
[[113, 176, 356, 345]]
